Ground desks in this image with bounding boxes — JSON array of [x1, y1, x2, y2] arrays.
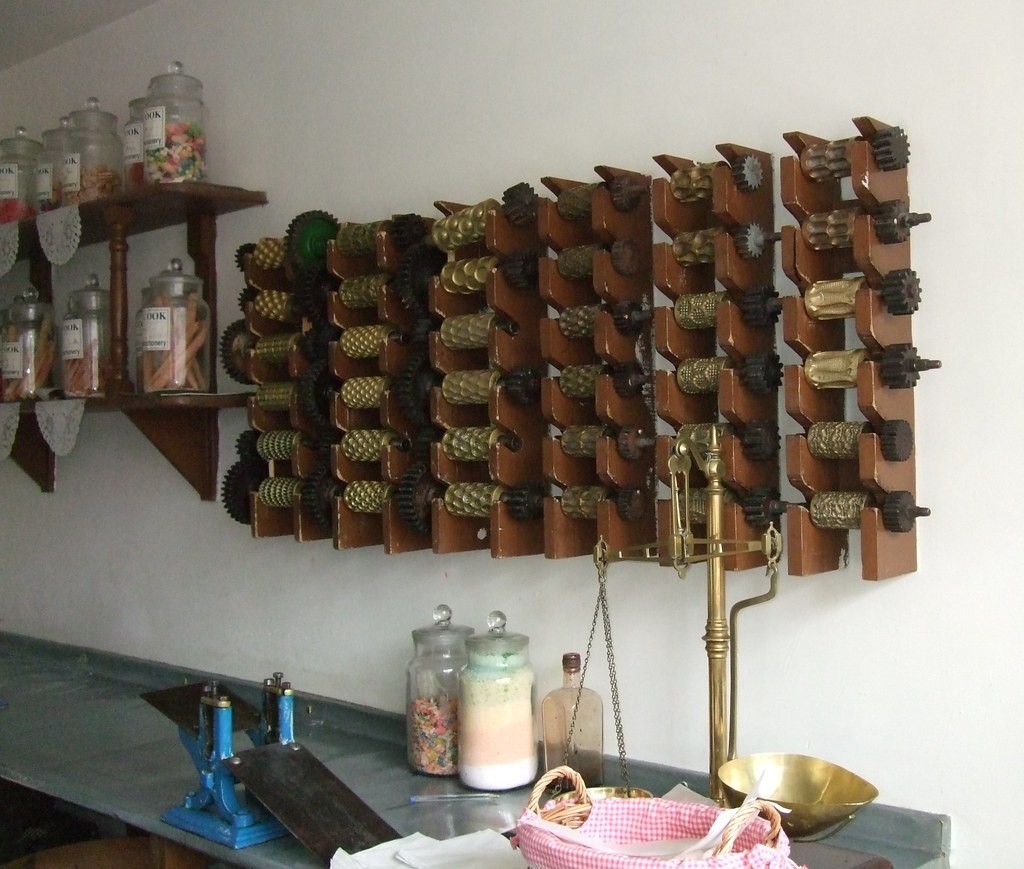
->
[[0, 632, 951, 869]]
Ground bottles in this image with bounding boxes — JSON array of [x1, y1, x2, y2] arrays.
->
[[135, 258, 212, 394], [0, 285, 51, 403], [405, 604, 475, 777], [0, 61, 208, 224], [457, 610, 539, 791], [542, 653, 603, 791], [59, 273, 111, 398]]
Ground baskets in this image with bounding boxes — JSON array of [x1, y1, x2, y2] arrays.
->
[[518, 765, 786, 869]]
